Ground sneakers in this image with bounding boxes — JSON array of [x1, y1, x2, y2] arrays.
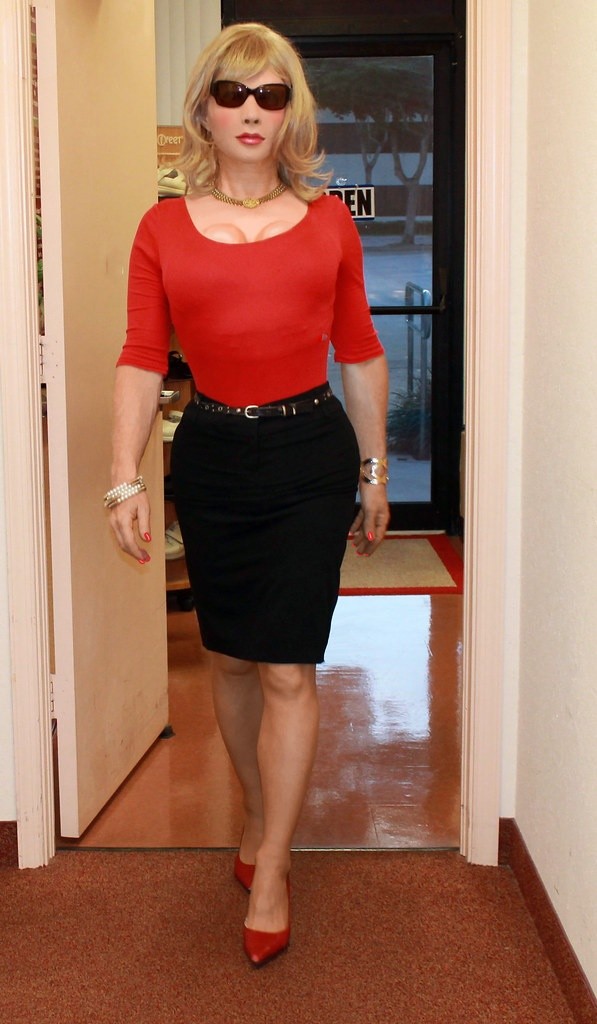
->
[[165, 520, 185, 559]]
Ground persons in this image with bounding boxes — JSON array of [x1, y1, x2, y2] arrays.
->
[[97, 21, 389, 966]]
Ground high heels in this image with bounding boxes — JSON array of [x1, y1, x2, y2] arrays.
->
[[243, 865, 294, 966], [235, 823, 254, 892]]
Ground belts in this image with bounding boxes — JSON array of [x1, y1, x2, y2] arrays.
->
[[191, 388, 332, 418]]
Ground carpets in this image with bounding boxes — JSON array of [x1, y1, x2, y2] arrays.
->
[[337, 530, 463, 596]]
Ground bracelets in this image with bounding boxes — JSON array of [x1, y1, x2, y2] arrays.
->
[[359, 457, 387, 487], [101, 475, 147, 508]]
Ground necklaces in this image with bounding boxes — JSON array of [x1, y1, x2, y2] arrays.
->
[[211, 180, 288, 208]]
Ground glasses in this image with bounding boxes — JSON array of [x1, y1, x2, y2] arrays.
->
[[209, 80, 293, 110]]
